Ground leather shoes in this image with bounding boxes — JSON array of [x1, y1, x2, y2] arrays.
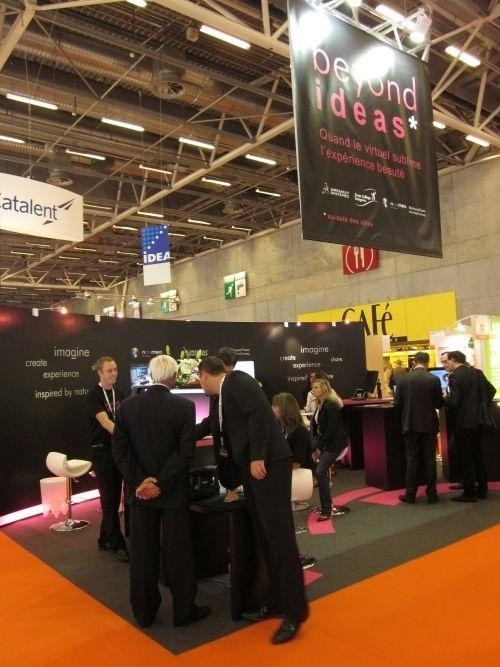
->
[[399, 495, 412, 503], [193, 606, 210, 619], [117, 550, 129, 562], [273, 617, 303, 644], [318, 516, 328, 521], [242, 606, 284, 621], [451, 493, 477, 502]]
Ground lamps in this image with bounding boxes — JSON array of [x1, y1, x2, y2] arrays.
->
[[100, 116, 144, 132], [0, 132, 287, 286], [346, 1, 481, 67], [6, 92, 59, 110]]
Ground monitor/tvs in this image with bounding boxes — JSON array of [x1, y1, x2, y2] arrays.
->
[[170, 389, 214, 440], [427, 367, 451, 399], [365, 371, 382, 398]]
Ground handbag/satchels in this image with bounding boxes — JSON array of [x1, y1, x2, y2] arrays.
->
[[191, 465, 219, 500], [478, 401, 497, 431]]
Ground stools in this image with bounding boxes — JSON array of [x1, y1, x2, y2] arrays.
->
[[315, 442, 350, 519]]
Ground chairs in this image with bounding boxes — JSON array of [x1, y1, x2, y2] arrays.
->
[[292, 468, 314, 511], [45, 450, 91, 531]]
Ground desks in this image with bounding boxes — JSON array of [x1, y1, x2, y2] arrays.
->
[[340, 397, 394, 470], [439, 399, 499, 482], [354, 404, 409, 489], [159, 493, 266, 621]]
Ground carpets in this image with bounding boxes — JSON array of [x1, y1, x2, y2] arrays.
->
[[0, 478, 500, 655]]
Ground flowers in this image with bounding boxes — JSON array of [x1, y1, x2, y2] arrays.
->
[[166, 344, 202, 389]]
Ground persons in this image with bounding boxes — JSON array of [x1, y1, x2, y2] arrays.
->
[[214, 346, 239, 373], [196, 354, 311, 643], [84, 354, 131, 564], [110, 353, 214, 629], [271, 350, 497, 522]]
